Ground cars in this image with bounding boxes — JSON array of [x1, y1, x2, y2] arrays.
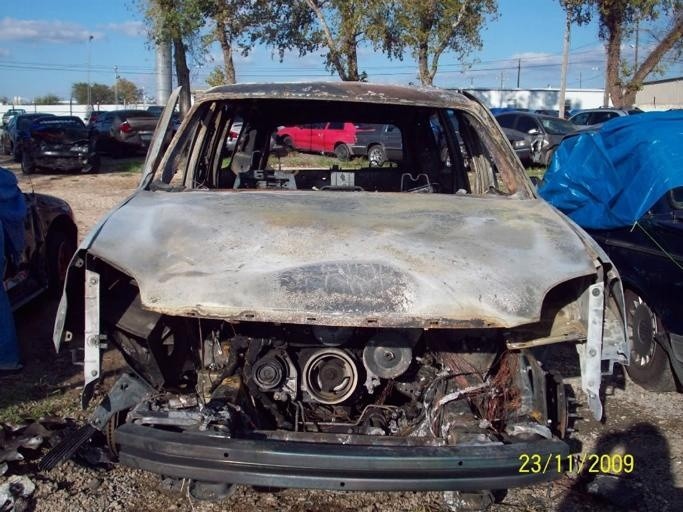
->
[[1, 106, 88, 195], [3, 194, 78, 319]]
